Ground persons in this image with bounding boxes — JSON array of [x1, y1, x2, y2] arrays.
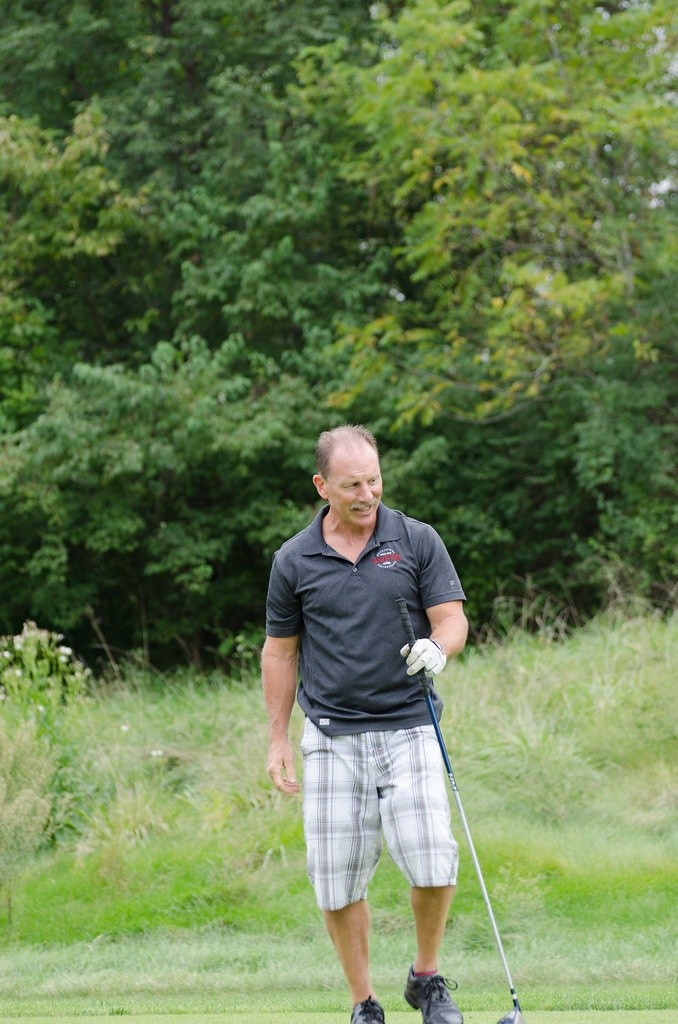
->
[[261, 425, 468, 1024]]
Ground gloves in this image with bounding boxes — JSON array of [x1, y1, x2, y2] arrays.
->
[[400, 638, 447, 678]]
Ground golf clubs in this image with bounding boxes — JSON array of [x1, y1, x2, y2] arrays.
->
[[395, 598, 527, 1024]]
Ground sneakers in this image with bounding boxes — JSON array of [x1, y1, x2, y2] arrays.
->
[[403, 964, 463, 1024], [350, 995, 384, 1024]]
